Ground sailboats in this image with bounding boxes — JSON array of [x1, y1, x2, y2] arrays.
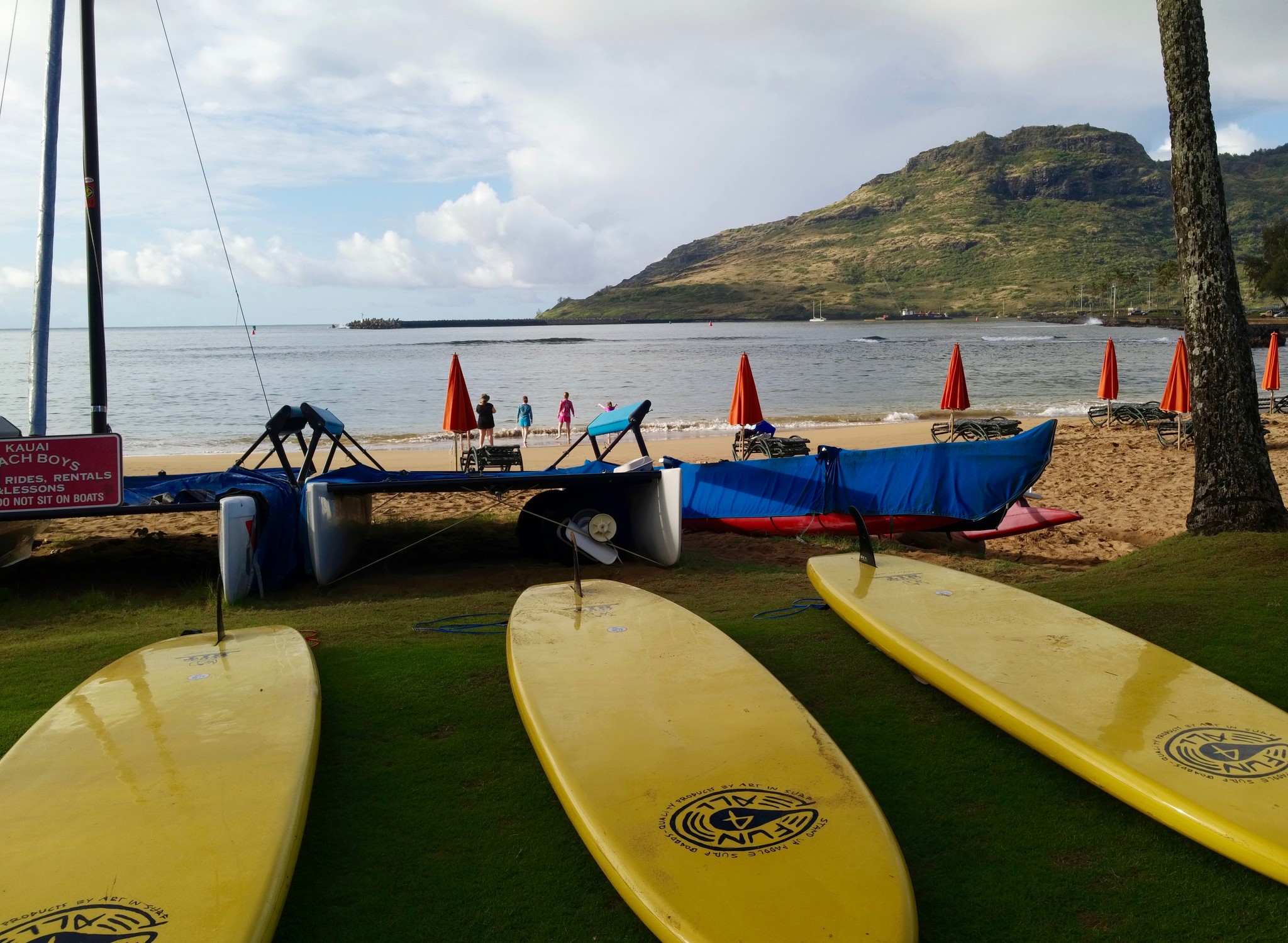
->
[[809, 300, 827, 322]]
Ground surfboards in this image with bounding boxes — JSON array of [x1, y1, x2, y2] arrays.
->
[[505, 531, 922, 943], [0, 572, 324, 943], [805, 505, 1288, 888]]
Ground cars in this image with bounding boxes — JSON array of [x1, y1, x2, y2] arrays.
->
[[1077, 313, 1086, 316], [1172, 310, 1182, 316], [1128, 311, 1142, 316]]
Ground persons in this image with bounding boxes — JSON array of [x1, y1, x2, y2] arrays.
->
[[476, 394, 496, 449], [517, 396, 533, 448], [599, 402, 619, 438], [555, 392, 574, 444]]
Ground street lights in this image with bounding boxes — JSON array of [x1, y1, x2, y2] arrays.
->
[[1079, 285, 1085, 311], [1145, 282, 1154, 311]]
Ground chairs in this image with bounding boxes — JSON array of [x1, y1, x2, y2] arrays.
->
[[1087, 401, 1194, 450], [1258, 395, 1288, 436], [460, 445, 523, 472], [931, 416, 1024, 441], [732, 434, 811, 462]]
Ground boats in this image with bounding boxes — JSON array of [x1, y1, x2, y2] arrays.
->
[[883, 316, 952, 320], [682, 416, 1060, 538]]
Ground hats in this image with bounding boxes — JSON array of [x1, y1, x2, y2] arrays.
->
[[479, 394, 490, 403]]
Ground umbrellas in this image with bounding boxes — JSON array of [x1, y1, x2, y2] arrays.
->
[[1159, 335, 1192, 450], [939, 341, 971, 442], [727, 351, 764, 461], [442, 352, 478, 471], [1097, 336, 1119, 429], [1261, 331, 1280, 414]]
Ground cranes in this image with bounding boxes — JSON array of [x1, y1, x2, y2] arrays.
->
[[882, 275, 903, 316]]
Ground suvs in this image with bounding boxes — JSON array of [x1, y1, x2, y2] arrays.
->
[[1142, 310, 1158, 316]]
